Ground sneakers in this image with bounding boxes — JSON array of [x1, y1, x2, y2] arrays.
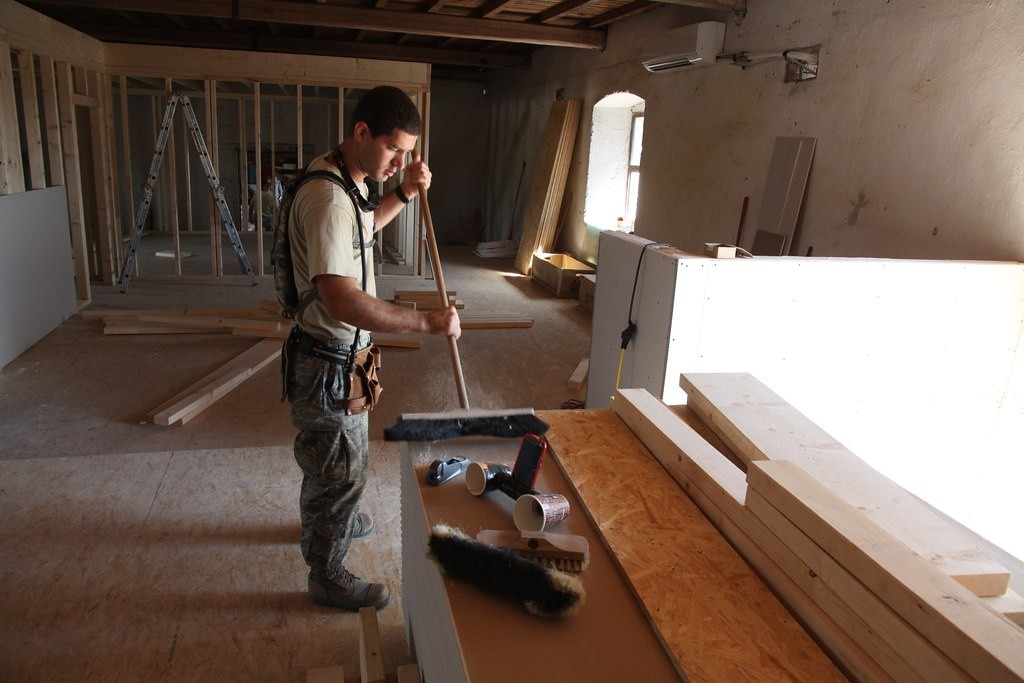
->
[[353, 512, 373, 538], [308, 566, 391, 610]]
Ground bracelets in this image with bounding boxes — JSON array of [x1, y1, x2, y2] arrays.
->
[[395, 186, 412, 205]]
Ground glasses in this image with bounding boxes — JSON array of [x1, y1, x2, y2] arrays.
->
[[357, 190, 380, 214]]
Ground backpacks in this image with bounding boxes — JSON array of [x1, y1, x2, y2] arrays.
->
[[270, 155, 360, 318]]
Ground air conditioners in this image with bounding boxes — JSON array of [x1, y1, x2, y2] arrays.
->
[[641, 21, 726, 73]]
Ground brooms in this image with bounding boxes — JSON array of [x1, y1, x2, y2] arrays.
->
[[383, 143, 551, 442]]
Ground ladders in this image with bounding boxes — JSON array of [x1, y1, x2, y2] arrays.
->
[[118, 89, 259, 295]]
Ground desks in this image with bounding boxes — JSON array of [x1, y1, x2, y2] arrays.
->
[[400, 407, 747, 683]]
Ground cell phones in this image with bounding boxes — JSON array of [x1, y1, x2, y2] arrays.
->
[[510, 433, 547, 490]]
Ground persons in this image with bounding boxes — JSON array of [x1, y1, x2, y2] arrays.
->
[[250, 170, 283, 232], [286, 86, 462, 612]]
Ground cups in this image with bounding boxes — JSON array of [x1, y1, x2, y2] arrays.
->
[[466, 462, 510, 495], [513, 494, 570, 534]]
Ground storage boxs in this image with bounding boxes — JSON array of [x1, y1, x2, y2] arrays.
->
[[531, 253, 594, 297], [579, 274, 596, 313]]
